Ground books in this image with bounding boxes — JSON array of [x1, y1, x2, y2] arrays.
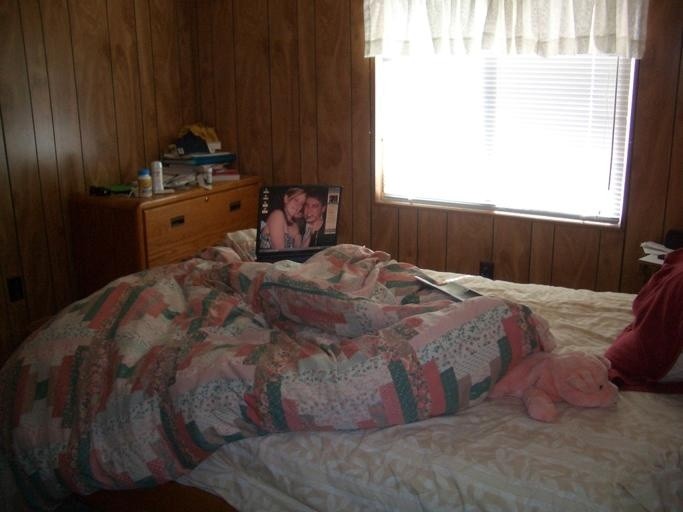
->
[[206, 166, 240, 181]]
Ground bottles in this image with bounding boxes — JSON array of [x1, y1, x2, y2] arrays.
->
[[135, 161, 163, 199]]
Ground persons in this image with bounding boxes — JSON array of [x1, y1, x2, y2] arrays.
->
[[259, 188, 326, 252]]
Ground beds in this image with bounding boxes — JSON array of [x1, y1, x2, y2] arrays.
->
[[70, 227, 682, 512]]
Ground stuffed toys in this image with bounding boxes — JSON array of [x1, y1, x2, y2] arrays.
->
[[488, 350, 620, 420]]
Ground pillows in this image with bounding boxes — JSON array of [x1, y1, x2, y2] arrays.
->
[[602, 248, 683, 392]]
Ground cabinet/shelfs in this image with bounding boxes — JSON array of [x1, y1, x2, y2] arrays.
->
[[65, 175, 265, 302]]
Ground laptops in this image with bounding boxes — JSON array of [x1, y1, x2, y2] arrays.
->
[[254, 184, 342, 264]]
